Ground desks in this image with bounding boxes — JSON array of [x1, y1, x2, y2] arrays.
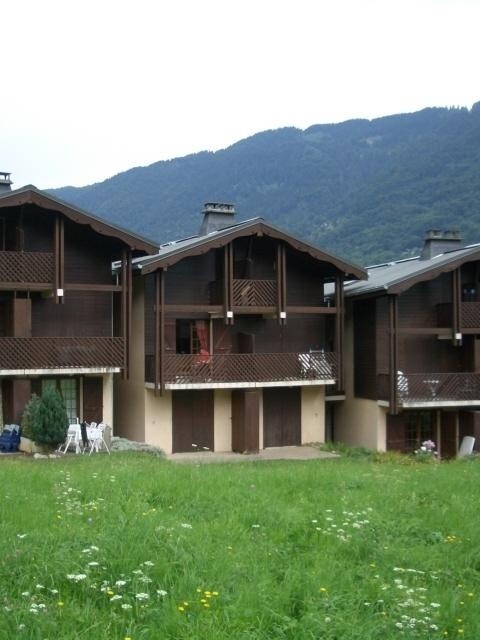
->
[[424, 378, 440, 398]]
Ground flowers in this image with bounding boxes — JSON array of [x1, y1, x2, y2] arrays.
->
[[422, 439, 436, 450]]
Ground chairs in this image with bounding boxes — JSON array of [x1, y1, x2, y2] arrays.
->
[[301, 349, 331, 380], [56, 422, 112, 456]]
